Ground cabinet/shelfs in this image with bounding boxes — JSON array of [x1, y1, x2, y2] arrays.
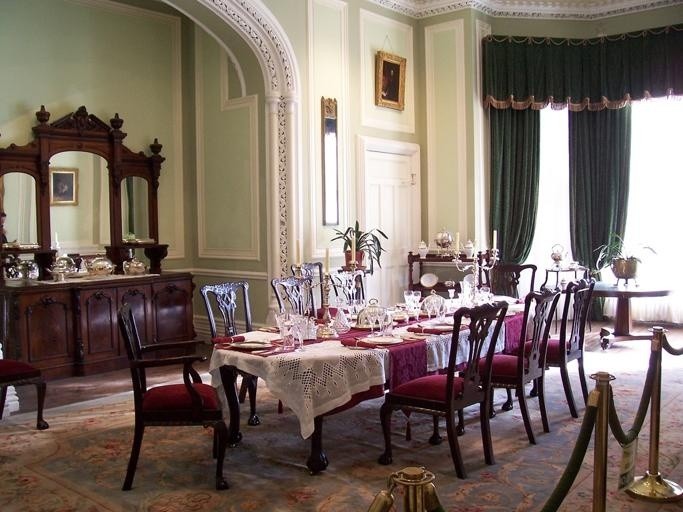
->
[[74, 276, 122, 379], [7, 282, 76, 382], [406, 252, 476, 299]]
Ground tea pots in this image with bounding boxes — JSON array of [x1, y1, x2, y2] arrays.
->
[[462, 239, 475, 258], [6, 255, 152, 281], [417, 239, 432, 260], [550, 242, 568, 264]]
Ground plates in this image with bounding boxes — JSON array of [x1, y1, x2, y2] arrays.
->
[[229, 282, 525, 354]]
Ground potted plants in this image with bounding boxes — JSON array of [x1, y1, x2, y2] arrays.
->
[[329, 216, 390, 276], [591, 233, 653, 280]]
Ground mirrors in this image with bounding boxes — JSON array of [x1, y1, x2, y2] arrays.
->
[[320, 94, 340, 227], [118, 136, 165, 271], [37, 102, 122, 268], [0, 136, 46, 270]]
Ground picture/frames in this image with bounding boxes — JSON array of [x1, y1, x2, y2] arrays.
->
[[48, 164, 79, 207], [371, 50, 408, 113]]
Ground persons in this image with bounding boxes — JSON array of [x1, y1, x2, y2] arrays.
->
[[380, 69, 398, 102], [0, 212, 8, 243]]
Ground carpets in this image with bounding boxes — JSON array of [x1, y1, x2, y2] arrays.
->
[[1, 366, 682, 512]]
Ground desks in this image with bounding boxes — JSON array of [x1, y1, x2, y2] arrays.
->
[[215, 294, 542, 476], [591, 283, 671, 351]]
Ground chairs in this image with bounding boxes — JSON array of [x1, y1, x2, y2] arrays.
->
[[483, 261, 537, 384], [455, 286, 563, 444], [116, 303, 227, 492], [380, 300, 510, 481], [200, 280, 293, 425], [500, 277, 596, 417], [270, 263, 366, 330]]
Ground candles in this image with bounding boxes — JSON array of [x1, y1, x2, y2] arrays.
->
[[473, 239, 478, 260], [350, 232, 355, 263], [492, 228, 497, 250], [294, 239, 301, 263], [454, 231, 459, 253], [325, 248, 330, 273]]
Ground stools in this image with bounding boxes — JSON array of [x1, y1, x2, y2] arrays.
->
[[1, 357, 49, 434]]
